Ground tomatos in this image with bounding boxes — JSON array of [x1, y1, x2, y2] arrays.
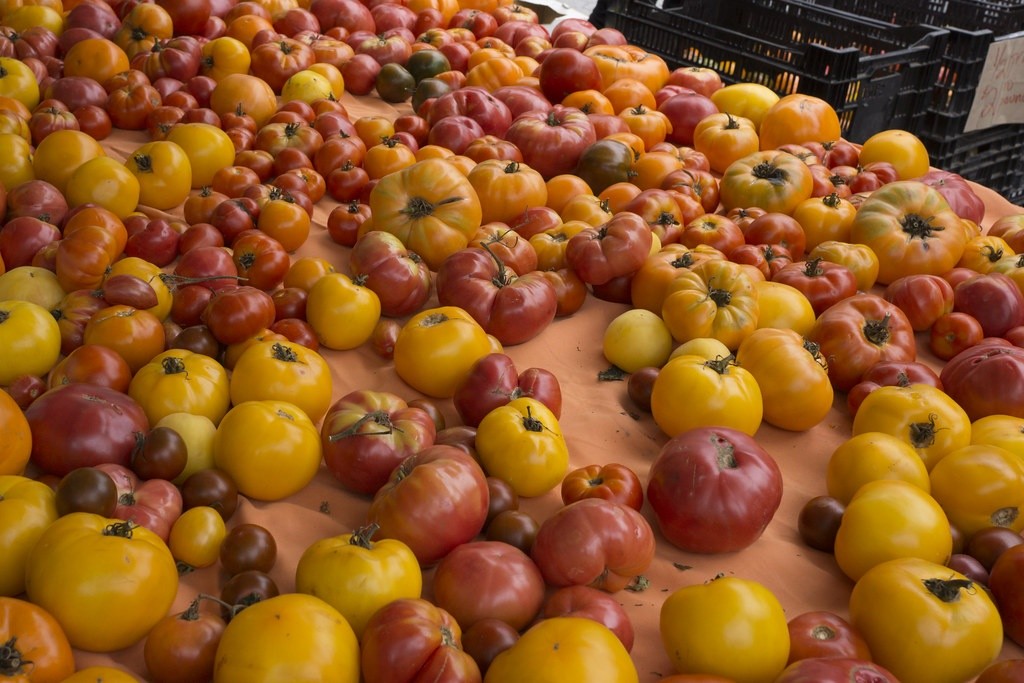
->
[[0, 0, 1024, 683]]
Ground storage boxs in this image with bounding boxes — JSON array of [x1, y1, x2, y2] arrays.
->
[[590, 0, 1024, 194]]
